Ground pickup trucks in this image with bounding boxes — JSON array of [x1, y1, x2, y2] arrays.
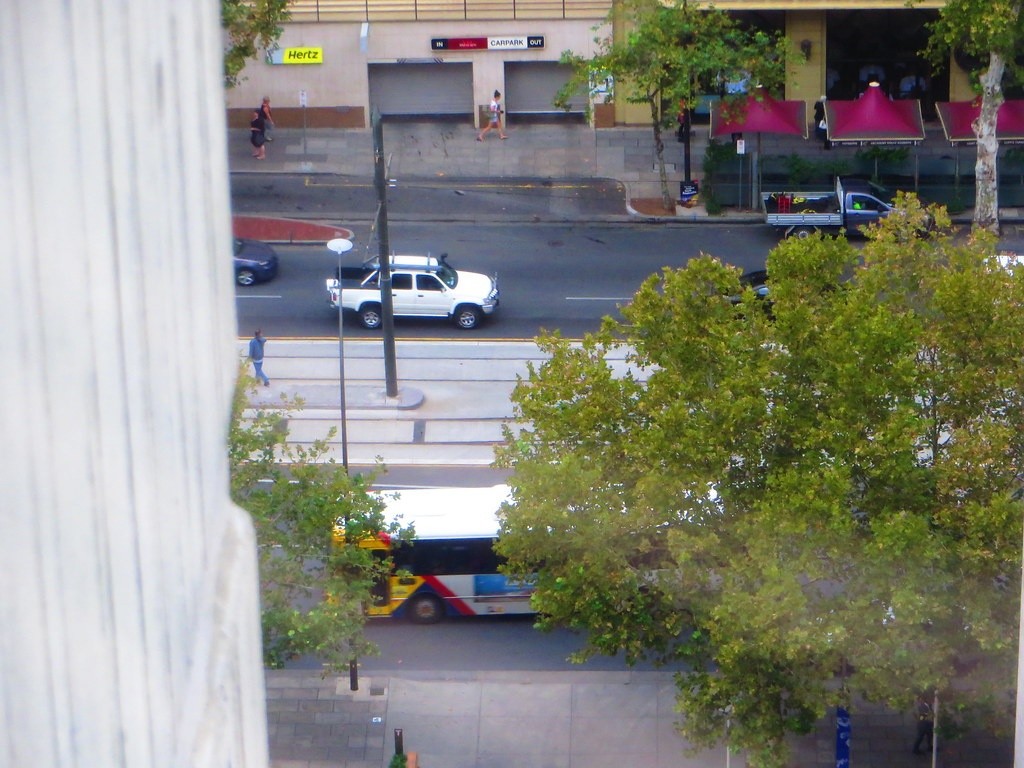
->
[[761, 173, 938, 243], [326, 253, 500, 330]]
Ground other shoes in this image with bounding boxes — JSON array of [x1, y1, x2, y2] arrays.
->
[[265, 136, 273, 141], [257, 155, 265, 159], [253, 153, 259, 156]]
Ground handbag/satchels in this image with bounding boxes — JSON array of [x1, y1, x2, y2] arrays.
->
[[487, 108, 494, 118], [255, 129, 265, 147]]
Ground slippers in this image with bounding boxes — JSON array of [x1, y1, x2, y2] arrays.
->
[[476, 136, 484, 142], [500, 135, 508, 139]]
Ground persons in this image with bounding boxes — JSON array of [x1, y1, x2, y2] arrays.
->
[[249, 328, 270, 386], [814, 96, 826, 134], [261, 96, 274, 141], [249, 111, 266, 160], [478, 90, 508, 141]]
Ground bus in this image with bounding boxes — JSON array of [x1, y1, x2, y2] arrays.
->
[[332, 473, 768, 628]]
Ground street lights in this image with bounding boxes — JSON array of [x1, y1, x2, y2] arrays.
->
[[326, 237, 357, 483]]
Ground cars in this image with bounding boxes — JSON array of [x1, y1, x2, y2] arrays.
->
[[232, 232, 278, 286], [722, 267, 852, 324]]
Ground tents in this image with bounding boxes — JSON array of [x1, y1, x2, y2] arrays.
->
[[710, 85, 810, 140], [824, 82, 927, 189], [935, 97, 1024, 189]]
